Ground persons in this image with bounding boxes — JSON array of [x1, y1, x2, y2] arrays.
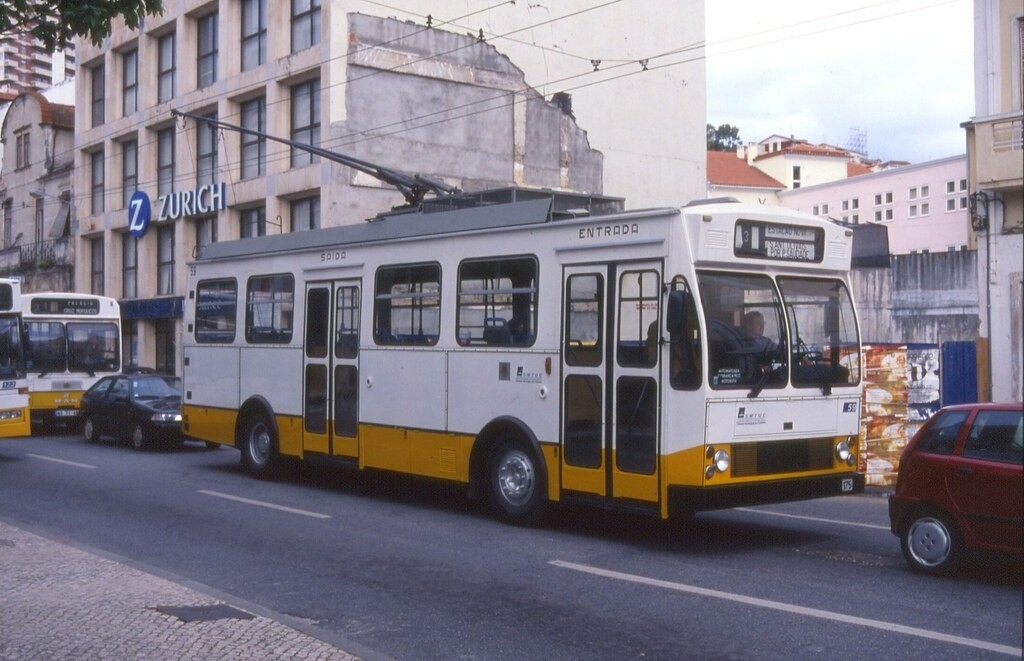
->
[[83, 330, 103, 363], [505, 317, 525, 343], [739, 312, 811, 384]]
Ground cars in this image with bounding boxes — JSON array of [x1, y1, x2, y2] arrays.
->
[[888, 403, 1024, 578], [78, 374, 181, 451]]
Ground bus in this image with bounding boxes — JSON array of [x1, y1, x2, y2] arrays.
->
[[0, 277, 32, 438], [179, 199, 868, 528], [14, 291, 124, 431]]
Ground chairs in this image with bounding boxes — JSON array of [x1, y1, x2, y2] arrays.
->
[[196, 315, 750, 391]]
